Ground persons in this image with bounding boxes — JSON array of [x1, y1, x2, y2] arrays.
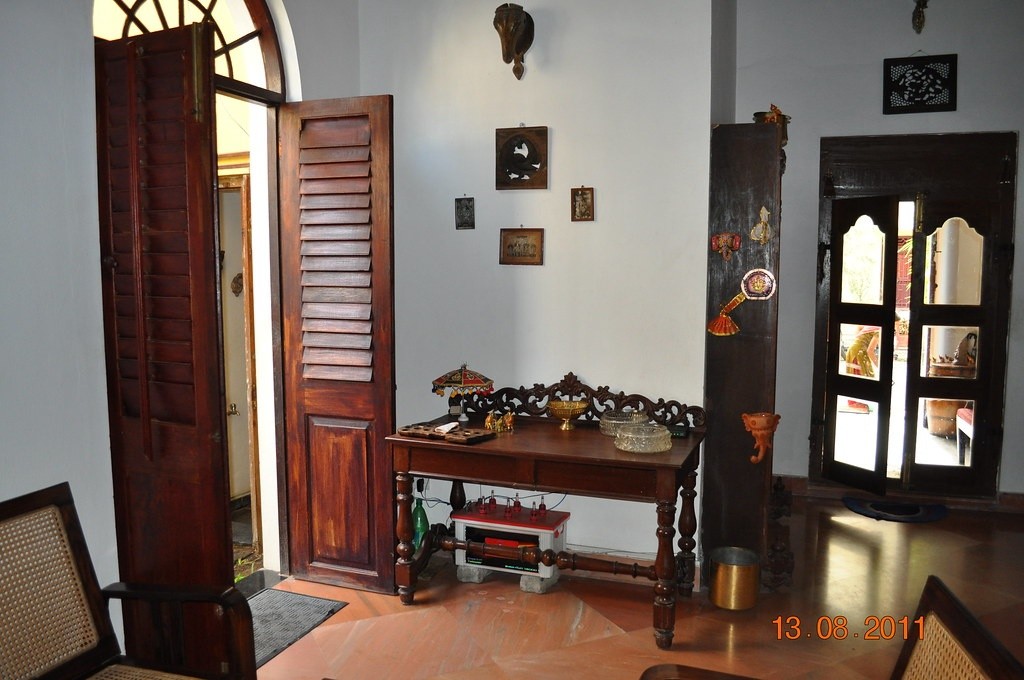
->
[[846, 312, 900, 412]]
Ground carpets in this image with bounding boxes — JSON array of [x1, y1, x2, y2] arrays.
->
[[246, 588, 348, 669]]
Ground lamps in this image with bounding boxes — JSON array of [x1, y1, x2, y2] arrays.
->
[[431, 363, 494, 422]]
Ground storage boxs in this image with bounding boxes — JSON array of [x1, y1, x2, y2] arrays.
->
[[450, 501, 570, 578]]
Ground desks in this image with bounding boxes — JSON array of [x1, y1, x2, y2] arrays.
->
[[384, 372, 710, 650]]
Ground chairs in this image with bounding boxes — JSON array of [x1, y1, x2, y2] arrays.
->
[[639, 576, 1024, 680], [0, 481, 258, 680]]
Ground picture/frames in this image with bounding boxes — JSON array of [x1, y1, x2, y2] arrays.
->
[[454, 198, 475, 230], [570, 187, 594, 222], [499, 228, 544, 266]]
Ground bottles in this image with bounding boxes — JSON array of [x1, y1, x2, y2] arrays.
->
[[412, 498, 429, 551]]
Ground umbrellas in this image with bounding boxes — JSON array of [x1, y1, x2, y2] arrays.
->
[[432, 362, 495, 426]]
